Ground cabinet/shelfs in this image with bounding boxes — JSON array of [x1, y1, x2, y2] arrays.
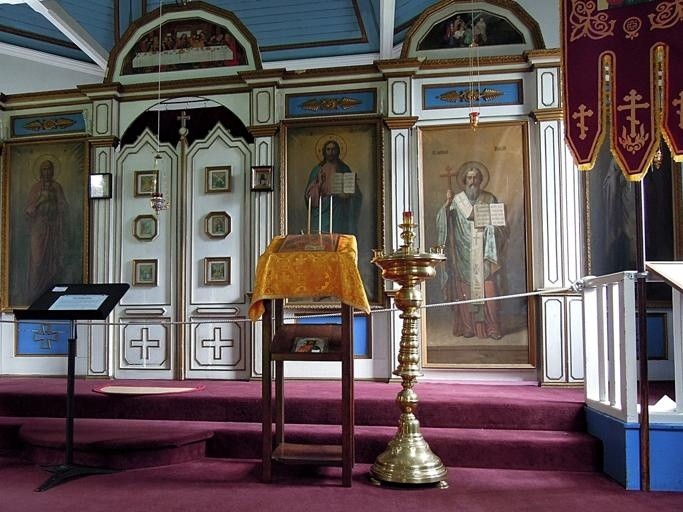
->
[[261, 234, 356, 488]]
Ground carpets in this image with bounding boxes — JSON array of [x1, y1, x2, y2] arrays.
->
[[92, 385, 205, 395]]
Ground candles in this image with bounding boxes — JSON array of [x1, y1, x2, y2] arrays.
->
[[306, 195, 333, 234]]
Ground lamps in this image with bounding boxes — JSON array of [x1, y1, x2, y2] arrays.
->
[[468, 0, 481, 133], [151, 1, 168, 216]]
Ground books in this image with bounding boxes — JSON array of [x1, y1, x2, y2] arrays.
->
[[329, 171, 357, 194], [473, 202, 506, 228]]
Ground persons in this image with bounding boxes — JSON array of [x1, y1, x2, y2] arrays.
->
[[445, 15, 487, 47], [305, 140, 362, 235], [435, 167, 503, 339], [137, 31, 240, 73], [27, 159, 69, 303]]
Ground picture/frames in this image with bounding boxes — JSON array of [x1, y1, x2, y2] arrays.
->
[[250, 166, 273, 192], [416, 120, 537, 369], [88, 173, 112, 199], [205, 166, 231, 286], [280, 113, 384, 311], [279, 233, 339, 252], [0, 134, 90, 314], [132, 170, 160, 287]]
